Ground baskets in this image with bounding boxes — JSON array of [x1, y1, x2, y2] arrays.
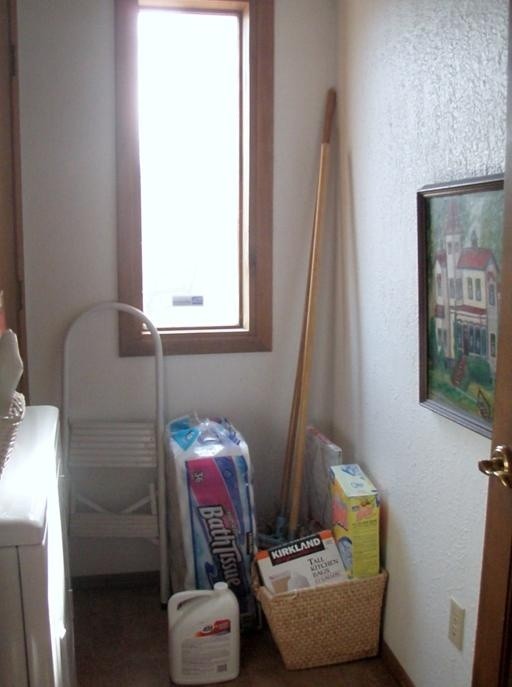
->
[[1, 392, 27, 472], [249, 560, 388, 670]]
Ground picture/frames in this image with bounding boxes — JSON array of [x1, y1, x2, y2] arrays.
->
[[416, 172, 504, 440]]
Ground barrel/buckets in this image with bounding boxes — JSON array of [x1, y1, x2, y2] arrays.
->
[[169, 584, 239, 684]]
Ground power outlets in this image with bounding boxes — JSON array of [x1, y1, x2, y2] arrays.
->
[[447, 597, 464, 652]]
